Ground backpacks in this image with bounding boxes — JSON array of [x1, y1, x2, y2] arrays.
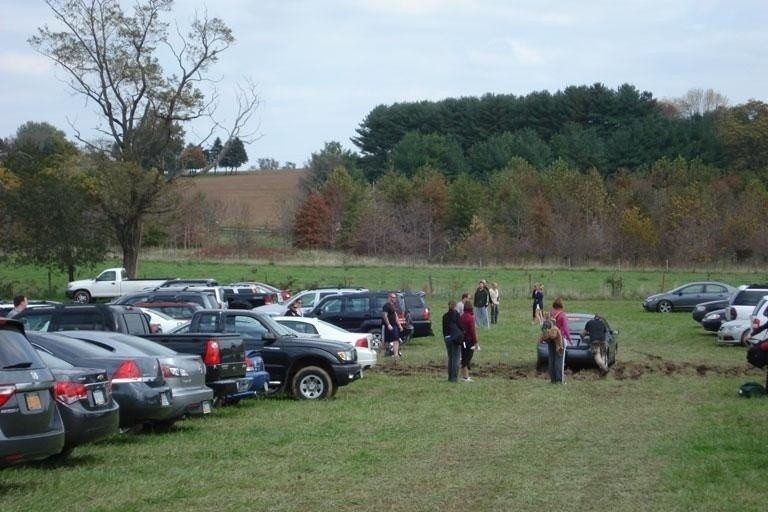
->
[[548, 311, 563, 326], [450, 311, 464, 345], [541, 311, 569, 357]]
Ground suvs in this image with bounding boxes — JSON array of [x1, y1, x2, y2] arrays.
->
[[725, 286, 768, 321], [751, 296, 768, 328], [252, 284, 369, 317], [0, 319, 66, 464], [191, 311, 363, 401], [134, 302, 204, 319], [145, 278, 216, 291], [221, 286, 256, 293], [112, 286, 222, 320]]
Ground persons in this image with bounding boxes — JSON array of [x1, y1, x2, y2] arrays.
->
[[534, 282, 544, 325], [454, 293, 473, 316], [295, 298, 303, 316], [579, 313, 613, 377], [5, 295, 28, 319], [474, 282, 490, 328], [489, 282, 500, 324], [443, 301, 466, 382], [460, 301, 477, 382], [546, 298, 574, 383], [284, 303, 301, 316], [531, 283, 544, 325], [382, 293, 403, 360], [482, 279, 489, 292]]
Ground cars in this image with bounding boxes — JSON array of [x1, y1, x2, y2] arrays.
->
[[222, 346, 271, 405], [643, 282, 737, 313], [228, 282, 290, 299], [535, 314, 619, 370], [400, 291, 431, 331], [167, 315, 377, 370], [49, 331, 214, 427], [141, 308, 192, 334], [702, 309, 727, 331], [25, 324, 174, 431], [718, 321, 754, 347], [33, 346, 121, 455], [225, 348, 269, 402], [693, 300, 730, 322]]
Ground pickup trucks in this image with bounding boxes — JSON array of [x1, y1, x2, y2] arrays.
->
[[66, 268, 180, 304], [11, 304, 253, 406], [223, 293, 278, 309]]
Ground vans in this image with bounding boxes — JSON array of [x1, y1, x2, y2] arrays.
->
[[304, 292, 407, 348]]
